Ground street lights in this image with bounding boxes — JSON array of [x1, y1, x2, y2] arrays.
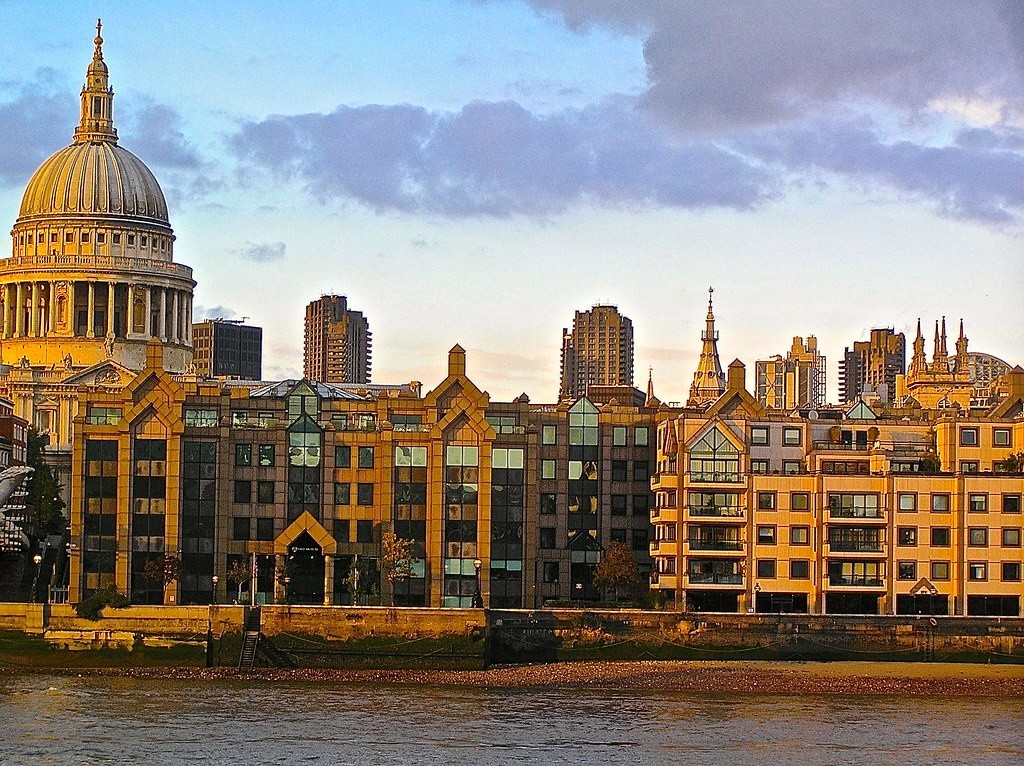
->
[[471, 559, 484, 608], [33, 554, 43, 603], [754, 583, 761, 613], [212, 575, 219, 605], [930, 586, 937, 616]]
